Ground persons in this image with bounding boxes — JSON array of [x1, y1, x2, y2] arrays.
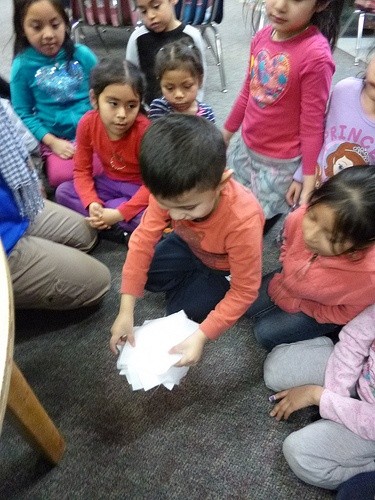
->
[[225, 0, 375, 489], [0, 0, 265, 369]]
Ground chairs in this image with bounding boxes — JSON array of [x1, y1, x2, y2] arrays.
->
[[72, 0, 375, 93]]
